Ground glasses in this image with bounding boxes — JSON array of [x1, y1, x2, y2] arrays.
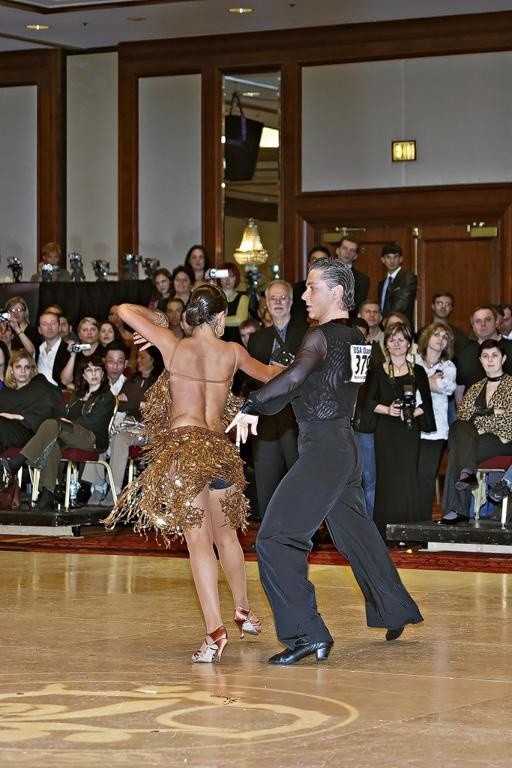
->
[[9, 308, 23, 312], [436, 301, 453, 306], [269, 295, 288, 300]]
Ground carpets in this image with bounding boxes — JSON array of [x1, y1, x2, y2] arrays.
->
[[1, 492, 512, 577]]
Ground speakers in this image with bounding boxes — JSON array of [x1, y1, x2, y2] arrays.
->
[[226, 114, 264, 181]]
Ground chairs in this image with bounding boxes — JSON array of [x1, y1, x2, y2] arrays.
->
[[1, 381, 511, 529]]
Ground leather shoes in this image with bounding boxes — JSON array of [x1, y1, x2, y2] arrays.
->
[[33, 492, 56, 513], [0, 456, 20, 476]]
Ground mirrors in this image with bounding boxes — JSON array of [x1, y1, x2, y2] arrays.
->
[[212, 54, 285, 293]]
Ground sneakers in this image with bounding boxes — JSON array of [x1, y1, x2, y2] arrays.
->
[[2, 491, 121, 513], [100, 489, 116, 507], [87, 487, 104, 507], [488, 481, 510, 501]]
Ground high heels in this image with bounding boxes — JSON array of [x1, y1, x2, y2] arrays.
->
[[387, 615, 425, 641], [268, 641, 335, 663], [191, 625, 227, 663], [454, 472, 480, 492], [433, 513, 472, 526], [233, 608, 262, 638]]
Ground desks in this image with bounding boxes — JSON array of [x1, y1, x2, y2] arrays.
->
[[1, 273, 158, 341]]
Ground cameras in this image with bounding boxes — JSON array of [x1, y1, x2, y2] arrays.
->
[[395, 383, 417, 431], [0, 312, 10, 320], [8, 254, 160, 277], [73, 344, 91, 352], [208, 268, 229, 281]]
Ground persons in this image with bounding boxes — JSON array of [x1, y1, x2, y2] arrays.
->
[[1, 235, 512, 548]]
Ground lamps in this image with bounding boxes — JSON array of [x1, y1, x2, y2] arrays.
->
[[230, 213, 271, 282]]
